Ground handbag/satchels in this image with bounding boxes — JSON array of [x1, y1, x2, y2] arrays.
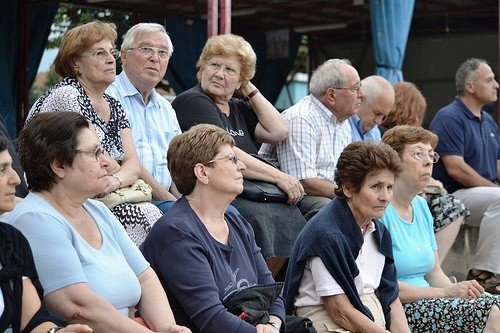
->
[[95, 178, 153, 211], [240, 154, 307, 205], [285, 315, 317, 333]]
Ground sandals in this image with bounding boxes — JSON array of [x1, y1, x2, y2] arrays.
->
[[466, 269, 500, 294]]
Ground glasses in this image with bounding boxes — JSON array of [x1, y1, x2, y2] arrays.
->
[[79, 49, 121, 60], [334, 83, 362, 92], [76, 147, 104, 161], [206, 61, 241, 76], [130, 47, 172, 61], [203, 153, 239, 166], [402, 151, 440, 163]]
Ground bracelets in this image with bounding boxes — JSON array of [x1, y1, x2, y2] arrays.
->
[[112, 173, 123, 190], [243, 88, 260, 102], [269, 321, 280, 331], [47, 326, 62, 333]]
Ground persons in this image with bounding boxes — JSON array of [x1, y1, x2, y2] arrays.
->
[[283, 139, 414, 333], [379, 124, 500, 333], [428, 57, 500, 295], [258, 59, 365, 223], [379, 81, 470, 268], [0, 20, 307, 333], [348, 76, 395, 144]]
